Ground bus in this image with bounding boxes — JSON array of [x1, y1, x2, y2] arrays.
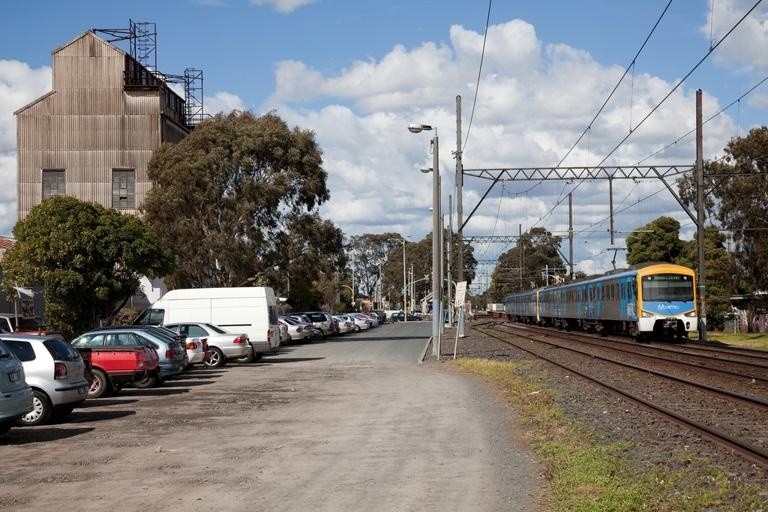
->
[[724, 291, 768, 318]]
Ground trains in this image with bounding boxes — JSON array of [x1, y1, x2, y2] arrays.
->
[[506, 262, 698, 342]]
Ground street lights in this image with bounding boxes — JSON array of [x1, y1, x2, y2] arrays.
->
[[403, 124, 444, 356]]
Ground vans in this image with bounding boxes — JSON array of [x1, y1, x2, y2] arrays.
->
[[129, 286, 280, 369]]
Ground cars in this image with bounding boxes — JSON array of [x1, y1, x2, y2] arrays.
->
[[279, 311, 386, 344], [0, 312, 252, 434], [390, 313, 422, 322]]
